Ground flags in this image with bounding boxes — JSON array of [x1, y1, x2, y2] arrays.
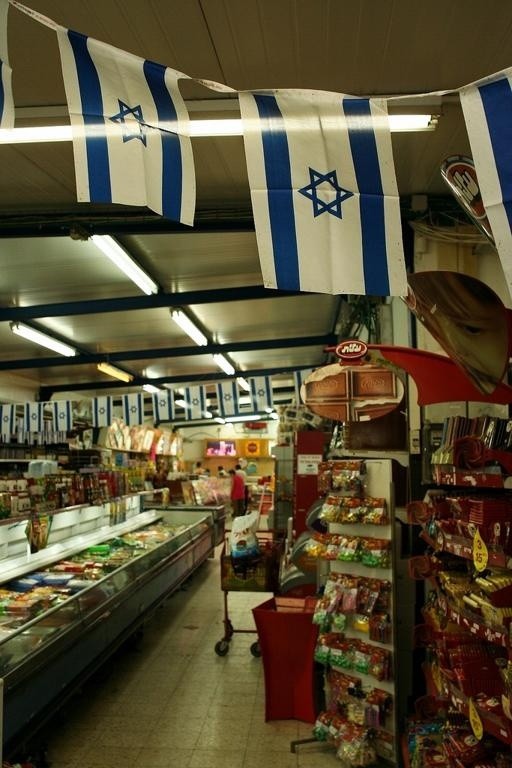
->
[[237, 89, 408, 297], [460, 68, 512, 299], [56, 25, 197, 228], [1, 368, 313, 445], [0, 1, 15, 129]]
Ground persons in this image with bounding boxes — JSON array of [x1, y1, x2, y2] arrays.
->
[[194, 462, 248, 517]]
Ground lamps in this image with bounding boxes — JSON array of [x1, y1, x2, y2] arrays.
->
[[169, 306, 208, 347], [96, 362, 134, 382], [90, 234, 158, 295], [214, 351, 279, 423], [143, 384, 214, 419], [10, 322, 76, 357], [0, 93, 443, 145]]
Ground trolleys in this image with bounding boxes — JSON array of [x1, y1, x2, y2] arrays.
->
[[215, 527, 286, 656]]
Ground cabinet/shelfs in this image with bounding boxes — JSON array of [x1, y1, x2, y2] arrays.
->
[[402, 459, 512, 768], [0, 493, 215, 744]]
